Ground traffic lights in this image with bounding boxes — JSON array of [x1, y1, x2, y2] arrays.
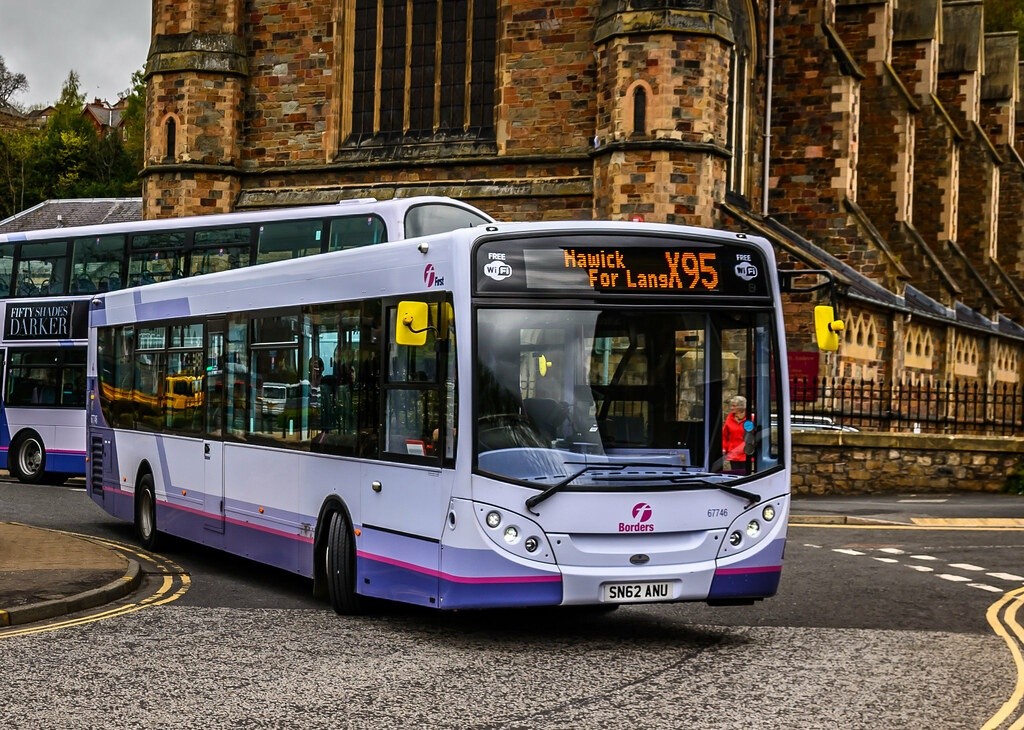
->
[[206, 358, 214, 372]]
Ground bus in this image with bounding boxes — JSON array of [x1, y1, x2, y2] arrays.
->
[[85, 221, 844, 614], [0, 196, 552, 484], [261, 382, 302, 417]]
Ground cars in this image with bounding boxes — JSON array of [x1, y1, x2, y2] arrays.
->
[[208, 363, 263, 390], [295, 331, 360, 378], [770, 413, 860, 432]]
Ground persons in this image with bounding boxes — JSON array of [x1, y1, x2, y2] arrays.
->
[[721, 396, 756, 474], [307, 356, 356, 384]]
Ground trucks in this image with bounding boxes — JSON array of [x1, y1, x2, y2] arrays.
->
[[100, 376, 204, 417]]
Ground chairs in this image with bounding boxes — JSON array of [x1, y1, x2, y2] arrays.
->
[[0, 268, 205, 299], [9, 376, 75, 406]]
